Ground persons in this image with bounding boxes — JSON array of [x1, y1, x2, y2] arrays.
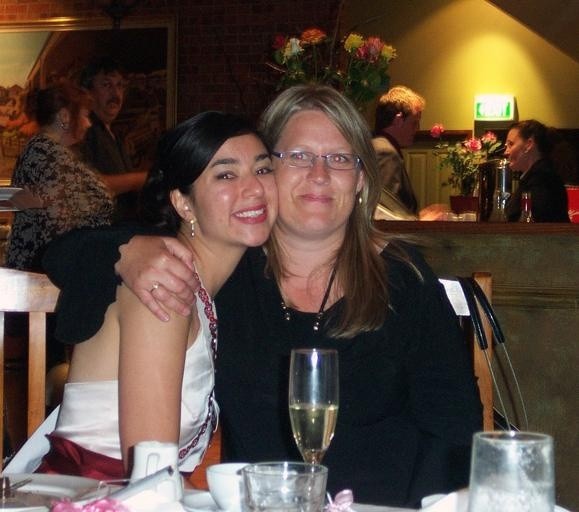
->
[[76, 55, 149, 222], [370, 85, 426, 214], [31, 109, 280, 491], [4, 80, 118, 453], [504, 119, 571, 223], [42, 81, 485, 509]]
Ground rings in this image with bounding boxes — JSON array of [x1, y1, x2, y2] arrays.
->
[[149, 284, 159, 293]]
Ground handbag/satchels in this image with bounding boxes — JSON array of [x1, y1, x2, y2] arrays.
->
[[466, 405, 519, 473]]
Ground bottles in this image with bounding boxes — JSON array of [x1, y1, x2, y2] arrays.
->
[[519, 191, 535, 222]]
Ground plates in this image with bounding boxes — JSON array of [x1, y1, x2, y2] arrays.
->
[[180, 490, 217, 511], [0, 472, 126, 511]]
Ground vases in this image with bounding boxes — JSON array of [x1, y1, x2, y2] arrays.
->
[[450, 196, 480, 222]]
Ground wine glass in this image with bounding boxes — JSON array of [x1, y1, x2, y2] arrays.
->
[[290, 349, 341, 471]]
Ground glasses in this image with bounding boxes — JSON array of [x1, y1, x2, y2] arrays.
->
[[268, 148, 362, 170]]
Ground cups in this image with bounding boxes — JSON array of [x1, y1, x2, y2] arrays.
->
[[242, 461, 329, 512], [441, 207, 477, 222], [203, 462, 250, 512], [470, 429, 557, 512]]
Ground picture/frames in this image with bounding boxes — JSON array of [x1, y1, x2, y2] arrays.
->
[[0, 8, 182, 186]]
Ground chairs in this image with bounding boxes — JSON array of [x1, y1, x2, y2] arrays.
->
[[0, 267, 60, 467], [435, 269, 495, 437]]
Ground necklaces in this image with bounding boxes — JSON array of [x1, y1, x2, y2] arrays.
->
[[282, 260, 338, 346]]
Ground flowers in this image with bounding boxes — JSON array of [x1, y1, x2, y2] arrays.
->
[[432, 123, 503, 195], [264, 25, 399, 113]]
